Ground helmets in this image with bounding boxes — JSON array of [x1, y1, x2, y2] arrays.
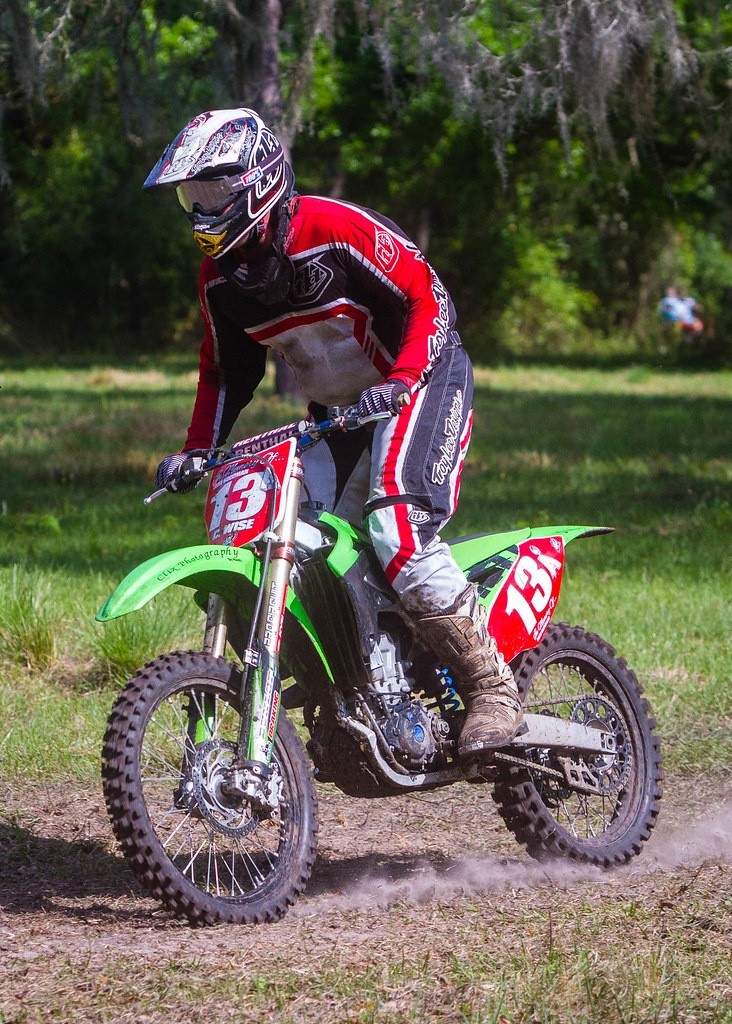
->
[[142, 107, 291, 260]]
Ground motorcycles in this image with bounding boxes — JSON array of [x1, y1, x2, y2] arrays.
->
[[92, 394, 666, 929]]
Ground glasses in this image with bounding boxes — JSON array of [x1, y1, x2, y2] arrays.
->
[[176, 143, 236, 217]]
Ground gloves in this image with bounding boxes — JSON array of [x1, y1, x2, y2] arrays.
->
[[358, 378, 411, 417], [154, 450, 207, 492]]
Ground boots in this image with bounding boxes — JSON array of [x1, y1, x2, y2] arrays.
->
[[412, 589, 529, 755]]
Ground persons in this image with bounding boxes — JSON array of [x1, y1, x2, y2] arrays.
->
[[142, 106, 531, 785], [659, 283, 707, 338]]
